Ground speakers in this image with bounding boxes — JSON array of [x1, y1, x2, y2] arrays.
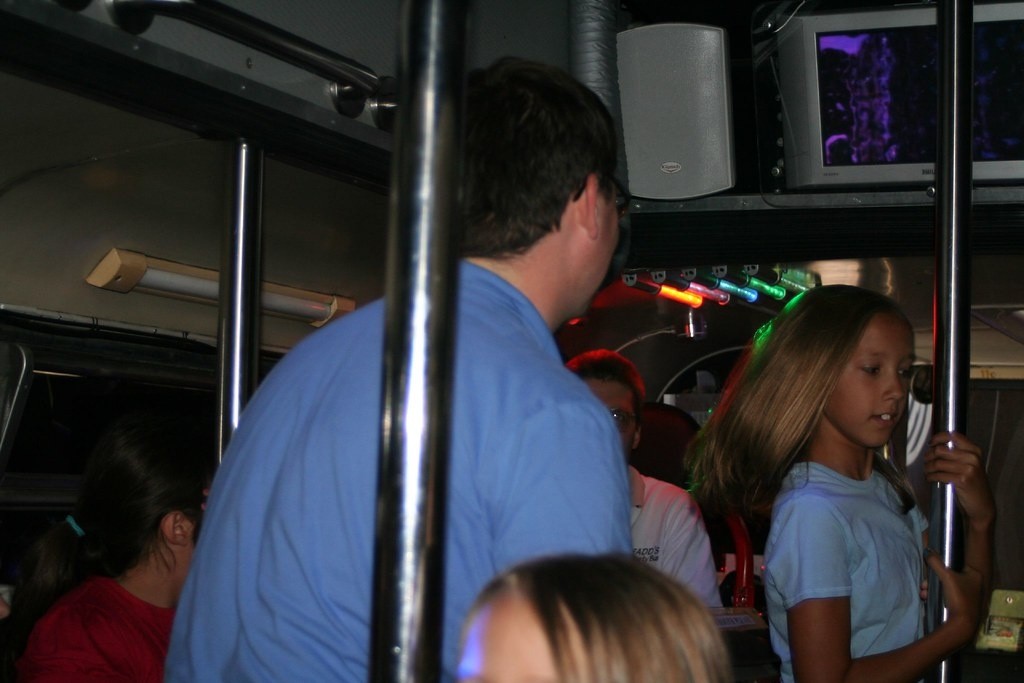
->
[[616, 22, 737, 200]]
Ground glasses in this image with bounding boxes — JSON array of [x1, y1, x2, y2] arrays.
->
[[608, 407, 639, 433]]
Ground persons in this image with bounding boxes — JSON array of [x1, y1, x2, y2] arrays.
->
[[0, 409, 218, 683], [684, 285, 997, 683], [164, 54, 732, 683]]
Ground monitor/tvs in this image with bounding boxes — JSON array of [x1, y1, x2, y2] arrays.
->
[[774, 0, 1024, 194]]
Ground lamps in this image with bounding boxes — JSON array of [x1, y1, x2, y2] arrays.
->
[[84, 249, 356, 328]]
